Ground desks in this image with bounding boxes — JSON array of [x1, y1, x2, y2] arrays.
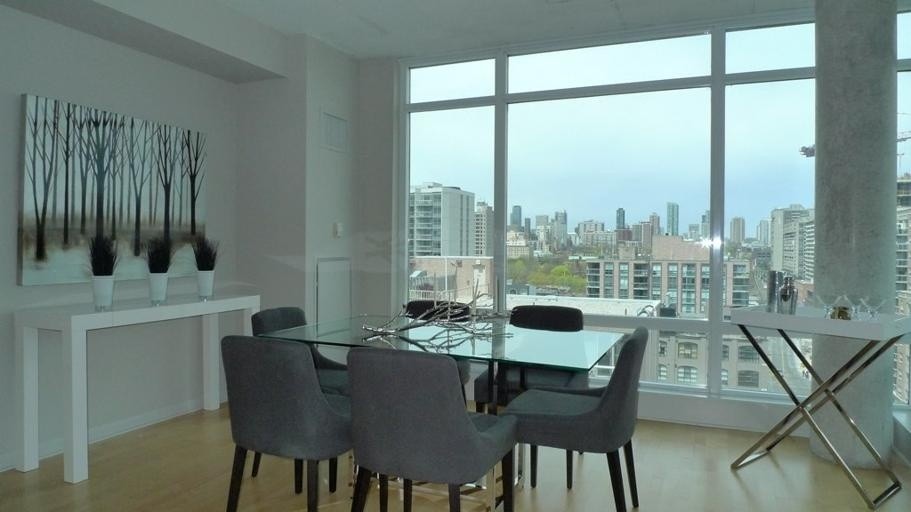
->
[[715, 299, 909, 512], [256, 313, 626, 511], [13, 290, 264, 485]]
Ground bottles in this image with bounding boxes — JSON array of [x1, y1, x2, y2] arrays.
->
[[780, 276, 798, 315]]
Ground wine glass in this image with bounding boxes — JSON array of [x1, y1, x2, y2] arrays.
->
[[816, 294, 887, 321]]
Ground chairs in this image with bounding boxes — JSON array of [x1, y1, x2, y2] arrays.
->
[[471, 303, 591, 459], [343, 338, 521, 512], [496, 324, 654, 512], [216, 327, 357, 512], [247, 303, 352, 497], [402, 298, 474, 404]]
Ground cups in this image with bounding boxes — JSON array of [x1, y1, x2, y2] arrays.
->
[[766, 269, 786, 312]]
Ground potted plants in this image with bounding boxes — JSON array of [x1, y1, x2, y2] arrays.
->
[[187, 232, 220, 297], [140, 234, 173, 303], [84, 236, 116, 310]]
